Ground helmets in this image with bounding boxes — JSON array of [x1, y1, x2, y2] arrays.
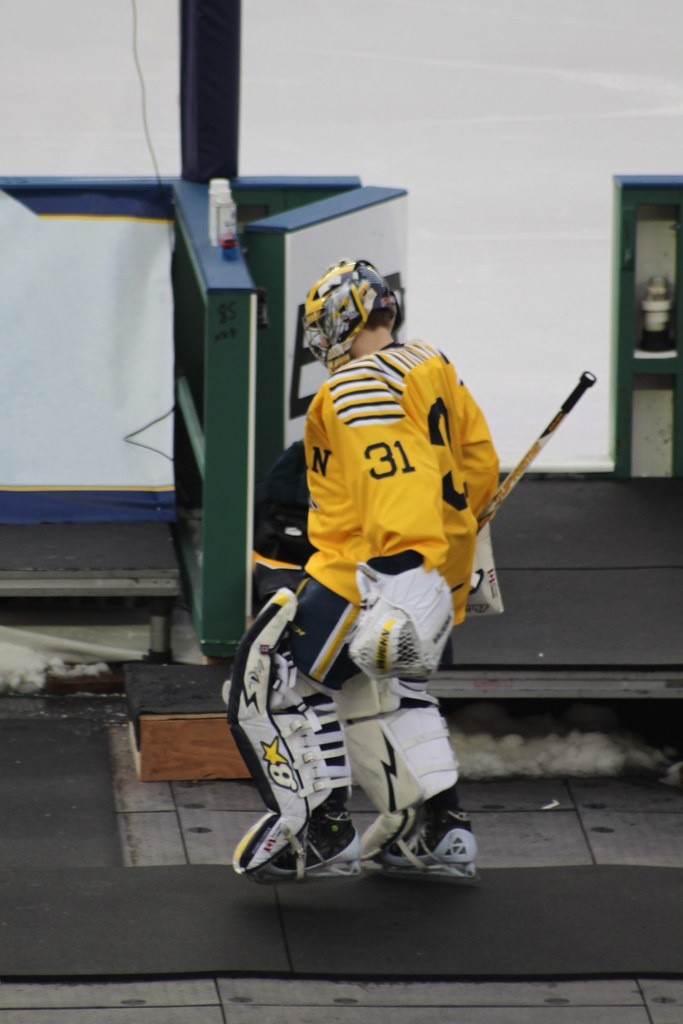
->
[[301, 259, 400, 371]]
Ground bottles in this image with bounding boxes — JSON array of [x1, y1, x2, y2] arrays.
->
[[213, 189, 239, 261], [209, 178, 231, 247]]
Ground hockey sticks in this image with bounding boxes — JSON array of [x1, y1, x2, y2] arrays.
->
[[474, 369, 599, 536]]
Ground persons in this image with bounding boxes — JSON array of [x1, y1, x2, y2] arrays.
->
[[222, 262, 498, 884]]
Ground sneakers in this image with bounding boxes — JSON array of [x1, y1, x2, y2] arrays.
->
[[265, 807, 361, 875], [380, 807, 484, 882]]
